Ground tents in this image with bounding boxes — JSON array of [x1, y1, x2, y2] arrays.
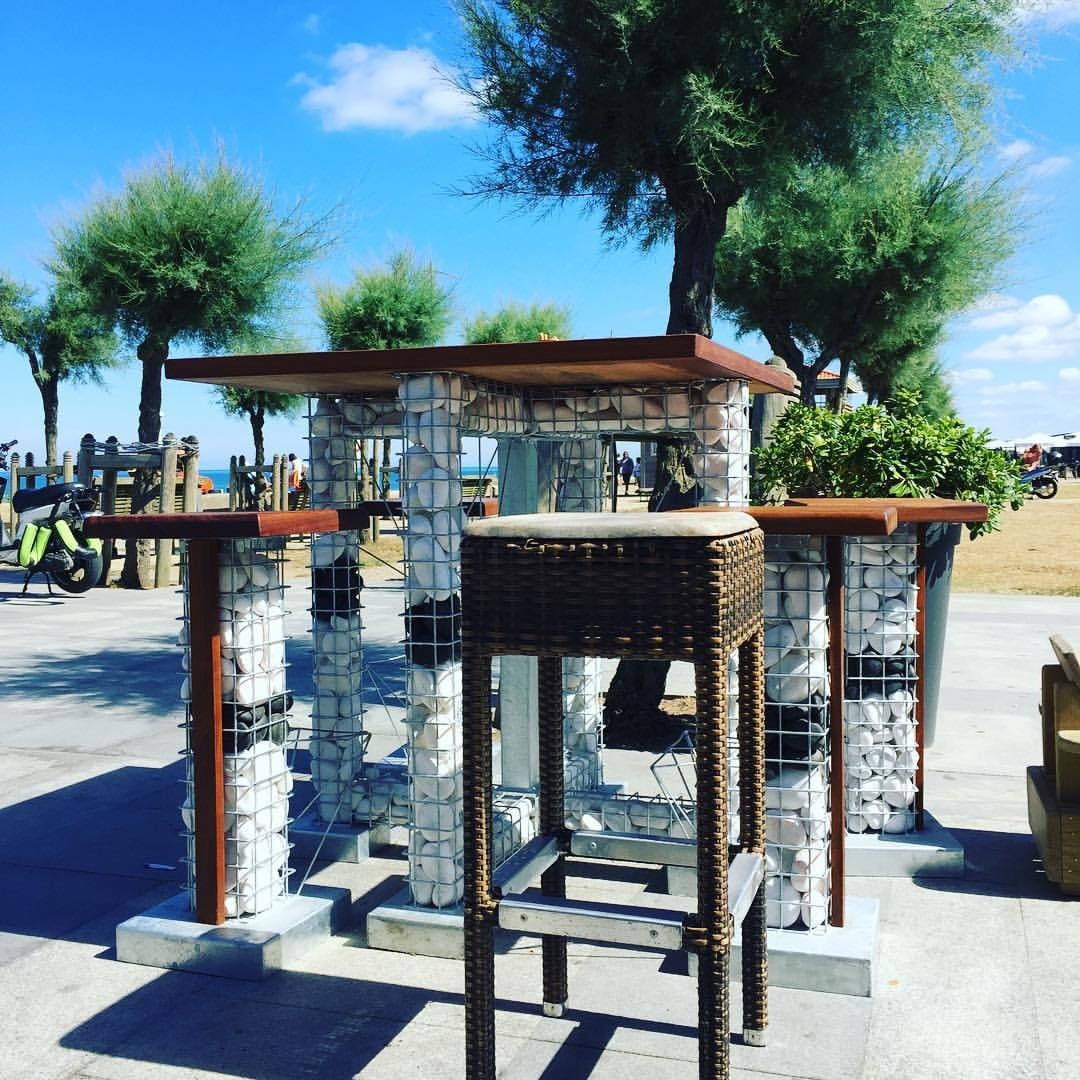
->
[[985, 432, 1080, 452]]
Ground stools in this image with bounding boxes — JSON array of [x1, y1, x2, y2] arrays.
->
[[460, 512, 769, 1080]]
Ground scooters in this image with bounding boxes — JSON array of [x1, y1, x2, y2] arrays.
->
[[0, 438, 106, 597], [1018, 451, 1063, 500]]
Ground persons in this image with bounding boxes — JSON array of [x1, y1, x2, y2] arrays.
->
[[237, 472, 256, 505], [289, 454, 307, 481], [617, 451, 634, 495], [1023, 444, 1041, 471], [1047, 447, 1063, 482], [634, 457, 641, 493]]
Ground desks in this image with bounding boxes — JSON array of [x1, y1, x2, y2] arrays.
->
[[82, 496, 986, 927]]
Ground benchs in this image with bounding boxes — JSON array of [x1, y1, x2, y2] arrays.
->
[[462, 478, 494, 497], [287, 490, 361, 541], [96, 477, 185, 516]]
